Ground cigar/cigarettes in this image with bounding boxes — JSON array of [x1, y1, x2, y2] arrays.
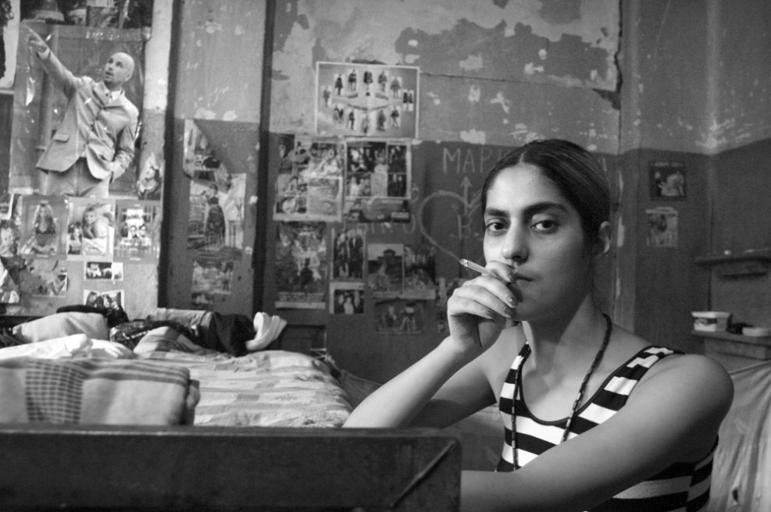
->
[[459, 256, 500, 281]]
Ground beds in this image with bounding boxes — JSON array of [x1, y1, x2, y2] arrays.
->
[[0, 317, 460, 512]]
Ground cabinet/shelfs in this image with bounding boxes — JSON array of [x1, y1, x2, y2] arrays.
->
[[689, 330, 771, 372]]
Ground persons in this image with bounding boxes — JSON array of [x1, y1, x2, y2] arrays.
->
[[80, 207, 107, 240], [68, 222, 84, 255], [29, 201, 54, 255], [16, 19, 145, 198], [342, 137, 737, 512], [185, 70, 432, 307]]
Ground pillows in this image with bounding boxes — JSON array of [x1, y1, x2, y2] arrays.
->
[[11, 312, 109, 343]]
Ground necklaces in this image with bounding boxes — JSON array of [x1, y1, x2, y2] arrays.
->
[[511, 312, 613, 473]]
